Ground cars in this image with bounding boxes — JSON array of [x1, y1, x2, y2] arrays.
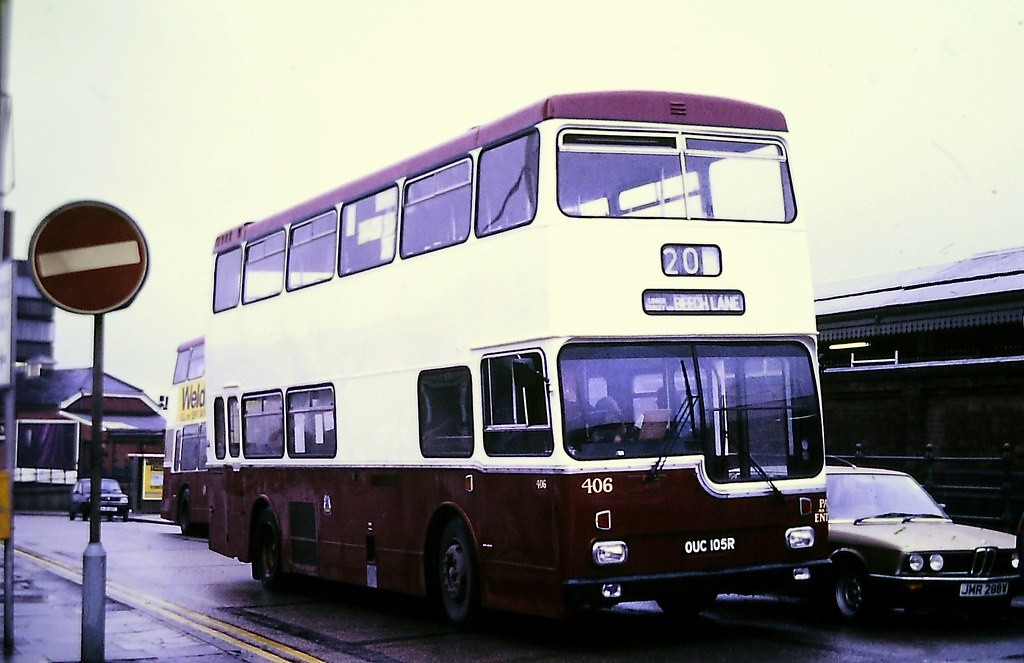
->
[[826, 465, 1021, 629]]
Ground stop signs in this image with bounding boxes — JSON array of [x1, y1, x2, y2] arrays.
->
[[26, 199, 153, 315]]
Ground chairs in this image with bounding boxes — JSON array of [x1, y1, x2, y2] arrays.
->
[[283, 428, 312, 454]]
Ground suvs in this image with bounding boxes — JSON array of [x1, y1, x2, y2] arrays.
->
[[68, 477, 129, 523]]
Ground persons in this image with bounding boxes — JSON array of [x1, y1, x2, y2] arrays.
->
[[592, 396, 627, 443]]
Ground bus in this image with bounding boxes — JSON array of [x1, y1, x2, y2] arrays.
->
[[203, 90, 833, 629], [158, 334, 206, 538], [584, 245, 1023, 532]]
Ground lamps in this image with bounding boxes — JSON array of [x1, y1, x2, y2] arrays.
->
[[829, 338, 872, 350]]
